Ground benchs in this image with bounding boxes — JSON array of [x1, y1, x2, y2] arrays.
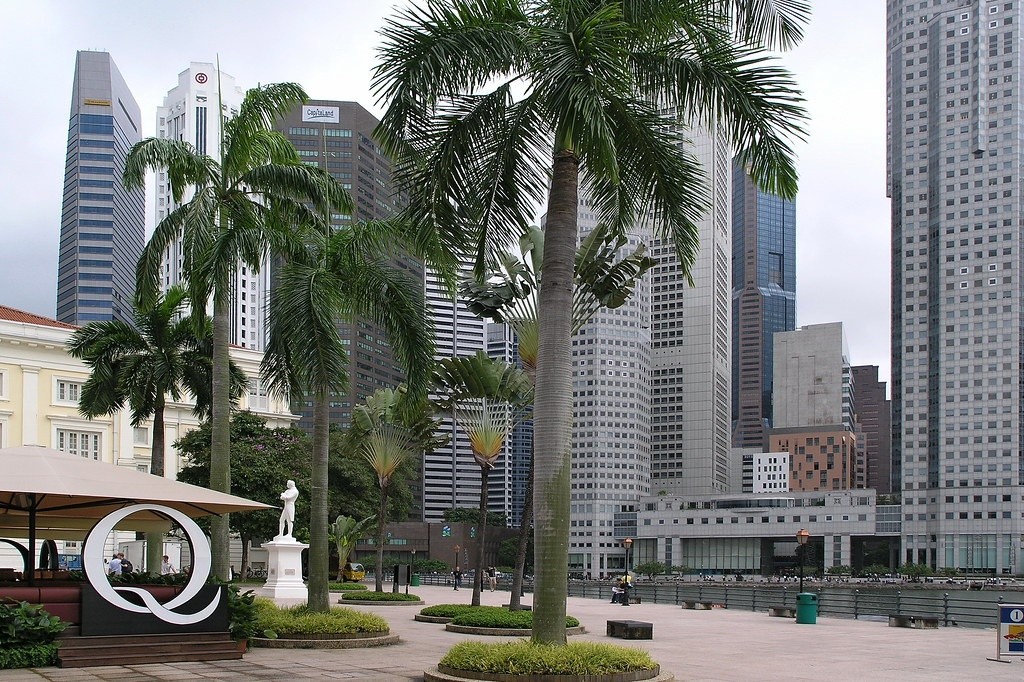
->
[[889, 614, 938, 629], [501, 603, 532, 611], [769, 606, 796, 618], [682, 600, 712, 610], [607, 621, 653, 639], [616, 593, 641, 604]]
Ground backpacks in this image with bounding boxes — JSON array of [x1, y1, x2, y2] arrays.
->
[[124, 560, 133, 573]]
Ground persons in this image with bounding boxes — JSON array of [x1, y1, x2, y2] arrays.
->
[[453, 566, 464, 590], [277, 480, 299, 537], [485, 564, 497, 592], [610, 571, 631, 603], [160, 555, 176, 574], [104, 553, 128, 575]]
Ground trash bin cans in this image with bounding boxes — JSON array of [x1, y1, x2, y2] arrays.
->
[[410, 574, 419, 586], [795, 592, 818, 624]]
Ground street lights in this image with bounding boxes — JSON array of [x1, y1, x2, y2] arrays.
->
[[796, 528, 810, 593], [621, 537, 632, 606], [411, 548, 416, 572], [454, 545, 461, 591]]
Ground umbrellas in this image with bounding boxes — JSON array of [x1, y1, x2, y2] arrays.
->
[[0, 447, 276, 588]]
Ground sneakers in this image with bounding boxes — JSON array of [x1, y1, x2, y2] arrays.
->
[[611, 601, 619, 604], [491, 590, 494, 592], [457, 589, 459, 591]]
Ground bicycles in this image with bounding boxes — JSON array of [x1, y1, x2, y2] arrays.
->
[[239, 566, 268, 578]]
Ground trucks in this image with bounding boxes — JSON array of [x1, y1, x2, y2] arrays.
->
[[330, 562, 366, 583]]
